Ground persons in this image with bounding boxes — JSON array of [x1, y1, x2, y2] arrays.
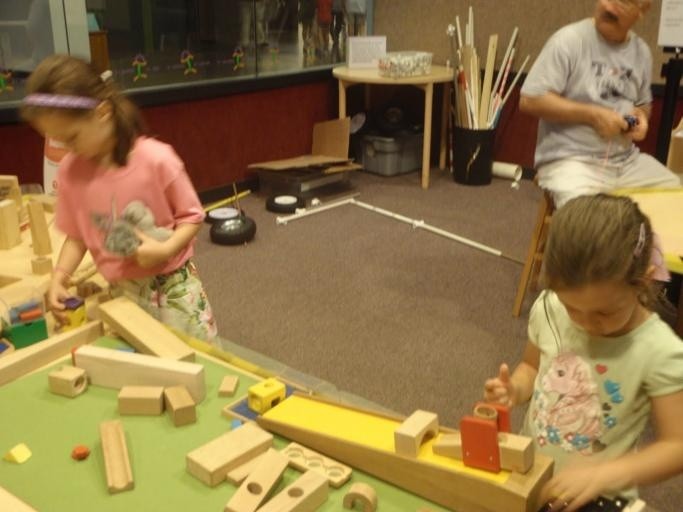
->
[[517, 1, 682, 238], [480, 191, 683, 511], [23, 50, 225, 349]]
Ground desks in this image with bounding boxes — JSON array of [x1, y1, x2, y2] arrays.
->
[[611, 187, 683, 275], [0, 313, 450, 510], [332, 63, 460, 190]]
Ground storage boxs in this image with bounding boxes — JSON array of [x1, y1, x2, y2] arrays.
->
[[361, 133, 423, 177]]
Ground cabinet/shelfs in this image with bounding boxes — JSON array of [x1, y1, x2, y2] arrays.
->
[[0, 1, 374, 204]]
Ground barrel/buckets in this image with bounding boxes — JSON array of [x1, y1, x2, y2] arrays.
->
[[452, 128, 496, 186]]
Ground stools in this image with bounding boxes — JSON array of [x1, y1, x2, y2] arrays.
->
[[511, 173, 558, 318]]
